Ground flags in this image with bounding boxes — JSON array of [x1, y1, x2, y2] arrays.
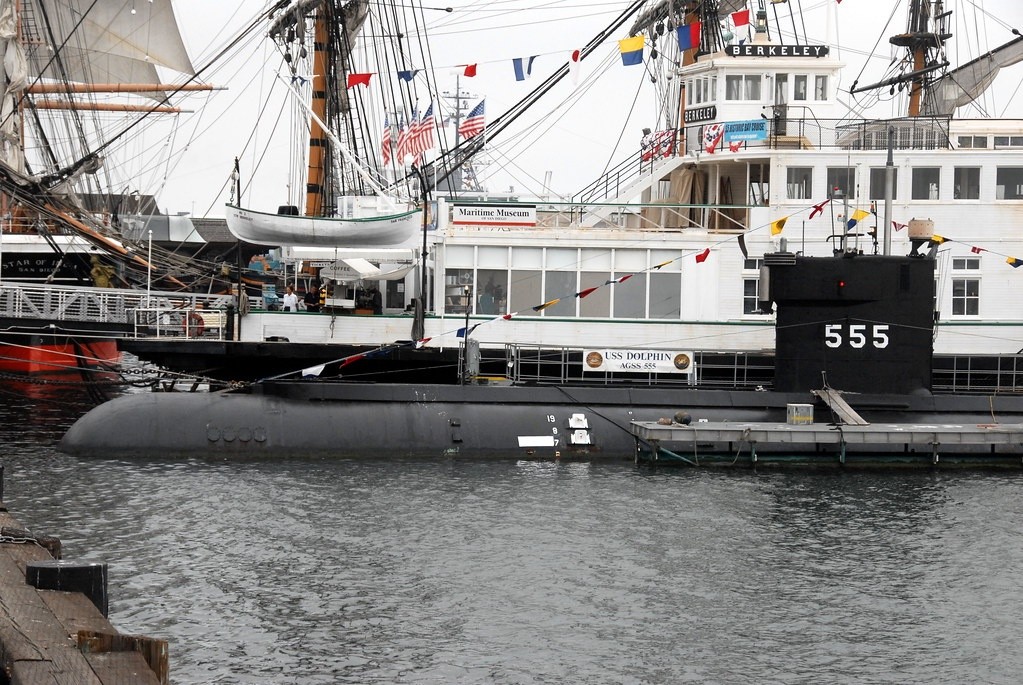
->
[[301, 199, 830, 376], [450, 63, 477, 77], [380, 99, 434, 169], [845, 209, 1023, 269], [457, 99, 485, 143], [397, 69, 425, 83], [677, 22, 701, 52], [731, 9, 749, 46], [618, 34, 644, 66], [346, 73, 377, 90], [641, 118, 767, 162], [289, 75, 320, 87], [437, 117, 449, 128]]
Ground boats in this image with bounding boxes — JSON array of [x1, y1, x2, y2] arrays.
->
[[55, 281, 1023, 461], [223, 157, 426, 246], [0, 341, 124, 396]]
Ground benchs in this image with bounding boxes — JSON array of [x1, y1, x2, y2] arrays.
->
[[194, 313, 227, 336]]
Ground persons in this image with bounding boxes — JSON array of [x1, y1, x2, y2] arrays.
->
[[367, 285, 382, 315], [282, 286, 299, 312], [304, 287, 321, 312]]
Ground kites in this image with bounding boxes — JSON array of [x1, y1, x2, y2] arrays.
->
[[563, 48, 581, 92], [513, 55, 539, 81]]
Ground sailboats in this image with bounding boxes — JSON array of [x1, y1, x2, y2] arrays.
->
[[0, 2, 1023, 403]]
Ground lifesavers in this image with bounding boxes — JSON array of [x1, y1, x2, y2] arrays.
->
[[182, 313, 204, 338]]
